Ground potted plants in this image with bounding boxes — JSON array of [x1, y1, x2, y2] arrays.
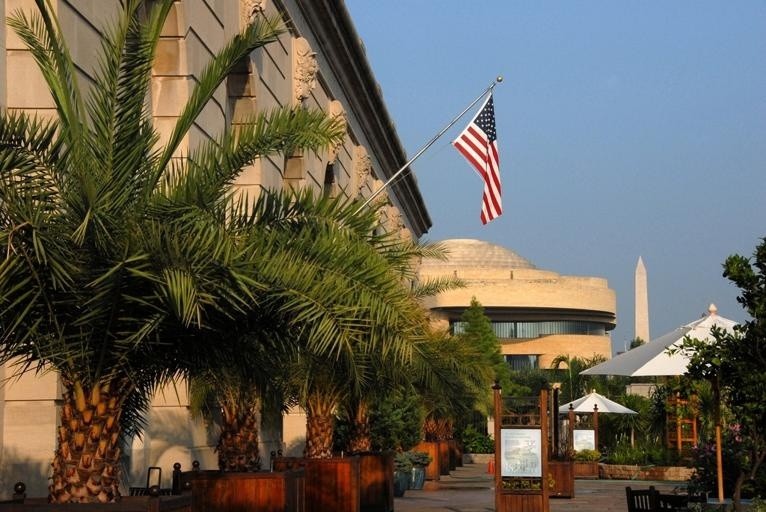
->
[[1, 4, 667, 511]]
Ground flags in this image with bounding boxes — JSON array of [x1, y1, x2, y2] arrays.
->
[[451, 90, 504, 225]]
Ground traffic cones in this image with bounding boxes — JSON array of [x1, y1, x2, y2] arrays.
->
[[488, 457, 494, 474]]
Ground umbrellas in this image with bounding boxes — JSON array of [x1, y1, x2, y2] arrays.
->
[[547, 388, 639, 415], [579, 303, 748, 502]]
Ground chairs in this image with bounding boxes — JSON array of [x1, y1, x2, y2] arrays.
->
[[626, 484, 706, 512]]
